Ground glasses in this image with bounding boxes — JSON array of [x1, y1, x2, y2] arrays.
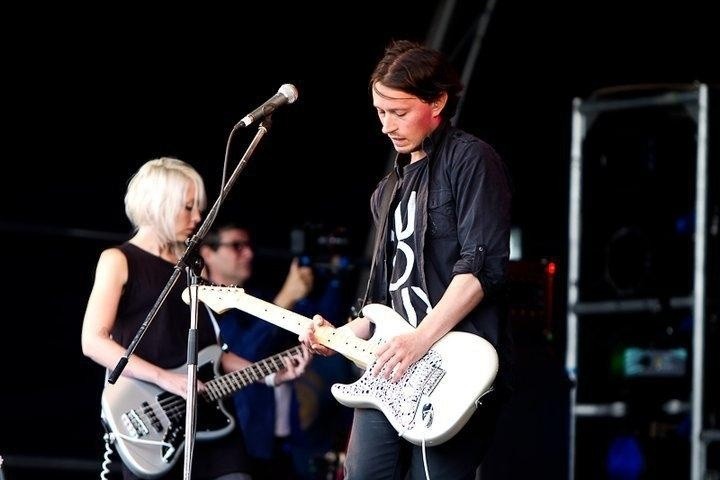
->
[[219, 241, 249, 251]]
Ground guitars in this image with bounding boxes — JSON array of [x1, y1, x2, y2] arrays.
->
[[180, 280, 499, 444], [102, 340, 309, 480]]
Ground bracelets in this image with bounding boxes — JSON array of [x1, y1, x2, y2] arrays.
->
[[264, 374, 278, 388]]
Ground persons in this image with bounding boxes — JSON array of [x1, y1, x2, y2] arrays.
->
[[81, 157, 313, 479], [196, 224, 315, 480], [298, 37, 514, 479]]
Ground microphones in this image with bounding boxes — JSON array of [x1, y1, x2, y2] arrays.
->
[[234, 83, 298, 129]]
